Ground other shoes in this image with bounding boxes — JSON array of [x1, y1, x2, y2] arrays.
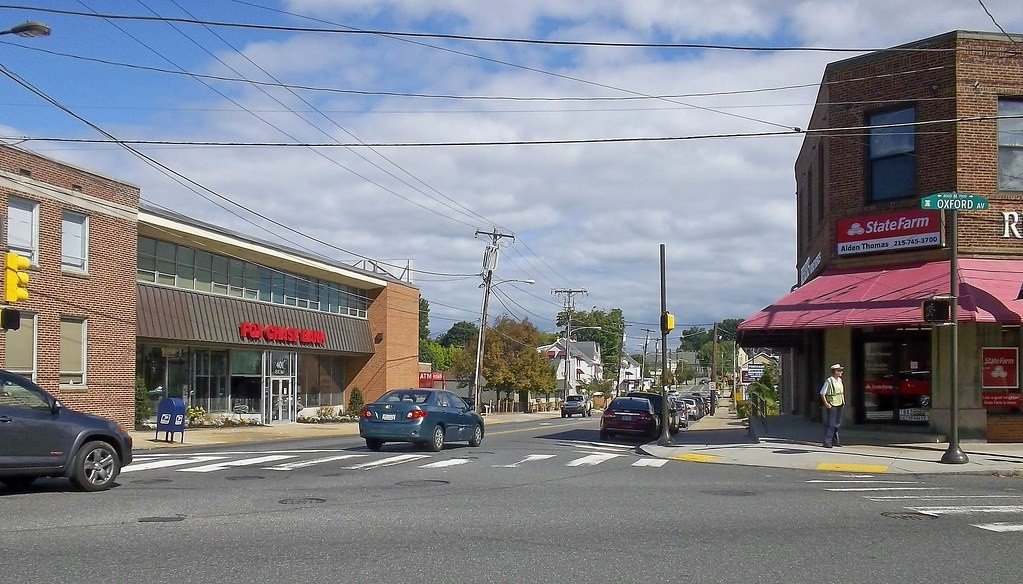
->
[[823, 443, 842, 448]]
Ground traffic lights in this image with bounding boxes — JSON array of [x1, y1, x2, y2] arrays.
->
[[617, 322, 671, 398], [5, 253, 29, 303]]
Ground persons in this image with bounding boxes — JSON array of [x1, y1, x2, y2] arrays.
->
[[820, 363, 846, 449]]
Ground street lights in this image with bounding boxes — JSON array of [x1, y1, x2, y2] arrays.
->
[[473, 228, 537, 420], [551, 287, 602, 401]]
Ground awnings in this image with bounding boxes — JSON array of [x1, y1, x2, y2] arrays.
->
[[734, 258, 1023, 348]]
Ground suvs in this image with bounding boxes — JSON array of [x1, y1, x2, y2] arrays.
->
[[562, 394, 591, 420], [625, 391, 681, 434]]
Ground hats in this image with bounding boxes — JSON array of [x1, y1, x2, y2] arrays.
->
[[830, 364, 844, 369]]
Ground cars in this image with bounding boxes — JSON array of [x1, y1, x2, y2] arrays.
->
[[640, 378, 656, 391], [659, 389, 723, 434], [698, 378, 709, 385], [360, 387, 486, 452], [600, 396, 662, 440], [0, 368, 134, 492], [619, 379, 639, 394]]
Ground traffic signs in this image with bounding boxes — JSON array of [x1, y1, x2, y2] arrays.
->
[[748, 363, 764, 379]]
